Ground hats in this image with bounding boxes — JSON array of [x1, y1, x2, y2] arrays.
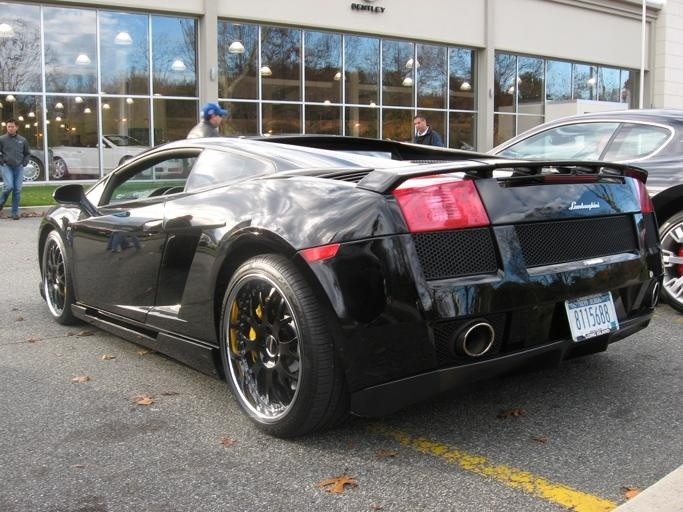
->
[[200, 102, 228, 118]]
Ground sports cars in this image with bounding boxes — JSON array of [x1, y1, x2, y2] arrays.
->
[[36, 131, 670, 437], [1, 131, 156, 181]]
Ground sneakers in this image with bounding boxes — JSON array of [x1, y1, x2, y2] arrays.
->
[[10, 211, 21, 221]]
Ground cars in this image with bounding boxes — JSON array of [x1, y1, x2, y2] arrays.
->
[[481, 110, 683, 321]]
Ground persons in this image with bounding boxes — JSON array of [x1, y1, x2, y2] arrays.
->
[[186, 101, 229, 139], [0, 118, 31, 221], [411, 115, 443, 147]]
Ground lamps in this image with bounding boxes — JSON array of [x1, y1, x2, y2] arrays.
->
[[460, 81, 471, 90], [334, 72, 347, 81], [406, 57, 421, 69], [402, 76, 415, 88], [370, 100, 377, 107], [76, 53, 90, 64], [171, 61, 186, 71], [1, 94, 136, 129], [1, 23, 13, 38], [114, 32, 133, 46], [228, 40, 246, 54], [261, 66, 272, 76], [324, 99, 331, 107], [353, 121, 361, 127]]
